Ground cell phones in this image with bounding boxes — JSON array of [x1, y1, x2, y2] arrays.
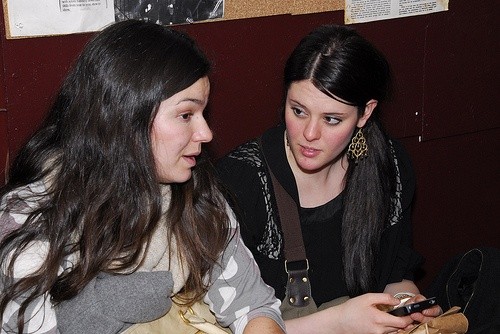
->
[[387, 298, 438, 318]]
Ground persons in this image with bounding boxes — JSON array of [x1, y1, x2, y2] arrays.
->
[[204, 24, 443, 334], [1, 20, 288, 334]]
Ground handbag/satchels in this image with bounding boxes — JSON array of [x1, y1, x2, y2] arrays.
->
[[425, 238, 500, 334]]
